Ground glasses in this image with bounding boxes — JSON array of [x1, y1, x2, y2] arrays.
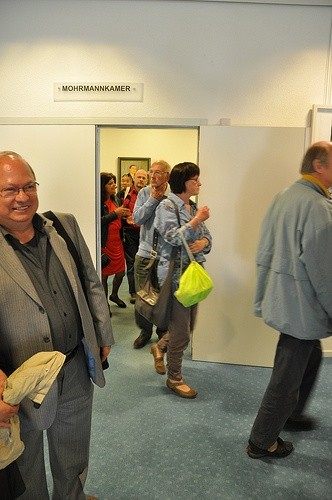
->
[[0, 182, 39, 198], [188, 177, 198, 182], [149, 170, 169, 175]]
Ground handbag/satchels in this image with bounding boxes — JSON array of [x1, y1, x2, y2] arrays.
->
[[173, 230, 215, 308], [134, 256, 181, 330], [100, 346, 109, 370], [101, 250, 111, 269]]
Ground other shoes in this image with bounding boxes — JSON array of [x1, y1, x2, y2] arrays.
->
[[167, 378, 197, 398], [86, 495, 98, 500], [109, 294, 127, 308], [151, 344, 166, 375], [130, 294, 136, 304], [108, 303, 112, 318]]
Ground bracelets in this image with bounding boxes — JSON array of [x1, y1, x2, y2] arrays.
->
[[194, 216, 200, 225]]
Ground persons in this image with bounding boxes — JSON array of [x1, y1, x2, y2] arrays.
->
[[132, 160, 171, 349], [0, 369, 26, 500], [100, 172, 131, 318], [116, 165, 149, 304], [0, 151, 115, 500], [151, 161, 212, 399], [245, 141, 332, 458]]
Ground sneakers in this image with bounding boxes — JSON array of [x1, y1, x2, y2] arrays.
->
[[134, 329, 152, 349], [159, 335, 167, 353], [282, 418, 314, 431], [248, 436, 293, 458]]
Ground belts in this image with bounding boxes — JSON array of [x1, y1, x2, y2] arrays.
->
[[64, 344, 79, 364]]
